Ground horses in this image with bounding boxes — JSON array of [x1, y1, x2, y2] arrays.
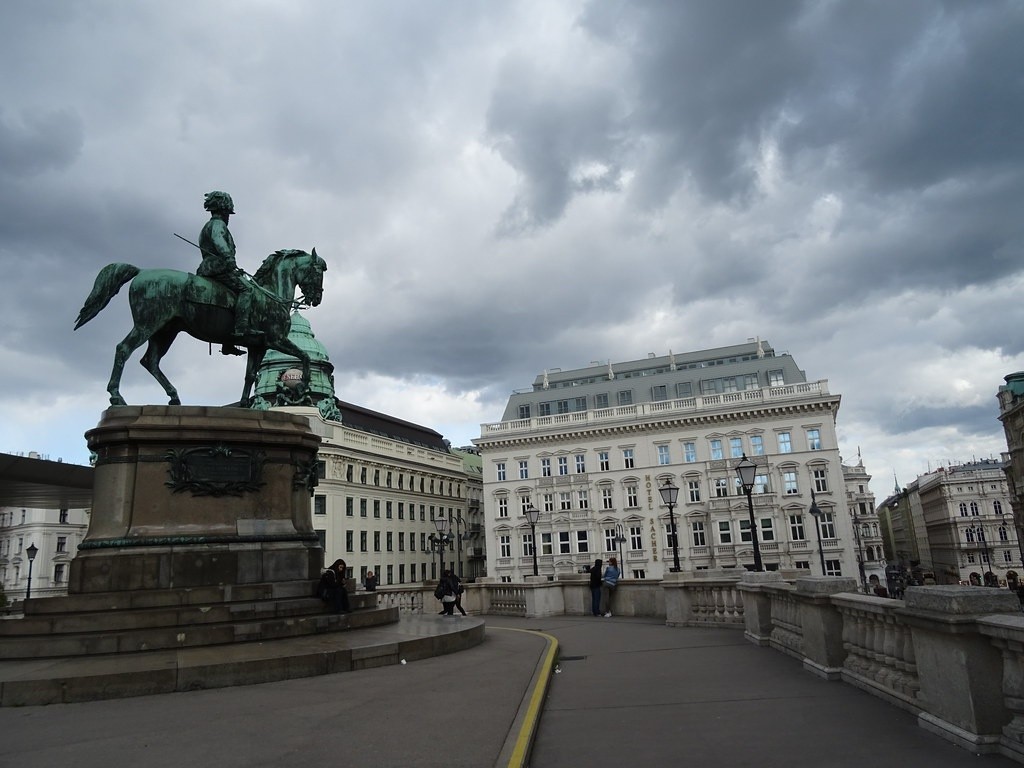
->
[[73, 247, 327, 408]]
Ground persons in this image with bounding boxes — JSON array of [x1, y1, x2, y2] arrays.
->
[[438, 570, 467, 616], [316, 559, 353, 614], [590, 558, 620, 617], [197, 191, 266, 356], [365, 571, 377, 592], [886, 573, 924, 600]]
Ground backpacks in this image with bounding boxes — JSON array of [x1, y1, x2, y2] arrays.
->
[[434, 583, 444, 602]]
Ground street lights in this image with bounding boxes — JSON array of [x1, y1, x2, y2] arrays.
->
[[524, 502, 541, 576], [1003, 512, 1024, 569], [810, 488, 827, 575], [447, 517, 468, 584], [971, 517, 995, 587], [734, 453, 765, 572], [614, 524, 628, 579], [658, 477, 681, 573], [853, 516, 870, 594], [428, 512, 449, 581], [26, 541, 39, 599]]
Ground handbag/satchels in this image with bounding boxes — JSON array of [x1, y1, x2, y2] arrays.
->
[[457, 582, 464, 594]]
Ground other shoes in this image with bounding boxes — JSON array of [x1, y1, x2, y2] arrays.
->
[[604, 612, 612, 618]]
